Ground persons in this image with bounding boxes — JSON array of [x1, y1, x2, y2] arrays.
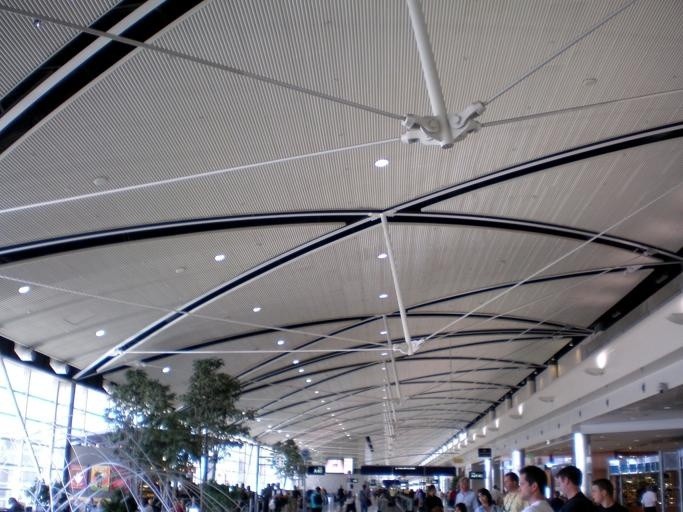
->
[[8, 498, 24, 512], [235, 465, 659, 511]]
